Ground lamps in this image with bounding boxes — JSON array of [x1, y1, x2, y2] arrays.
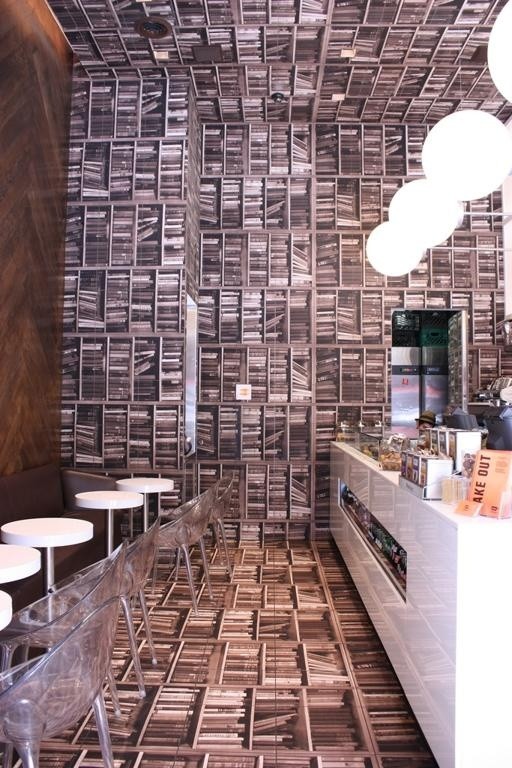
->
[[487, 1, 511, 104], [365, 222, 424, 276], [421, 109, 511, 203], [389, 179, 464, 249]]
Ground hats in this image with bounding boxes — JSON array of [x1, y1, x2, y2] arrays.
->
[[414, 410, 439, 426]]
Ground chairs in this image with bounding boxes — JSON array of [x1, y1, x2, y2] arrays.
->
[[0, 464, 234, 768]]
